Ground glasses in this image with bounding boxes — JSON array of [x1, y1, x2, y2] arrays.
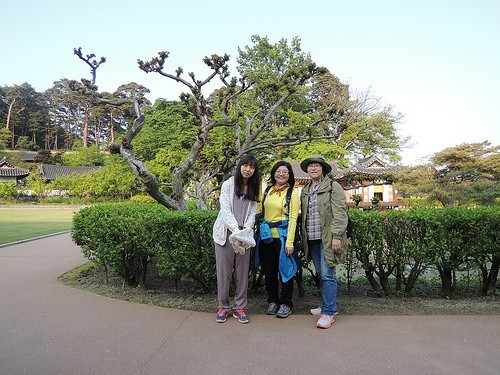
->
[[276, 170, 288, 175]]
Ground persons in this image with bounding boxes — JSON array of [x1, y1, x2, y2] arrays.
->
[[300, 157, 348, 329], [256, 161, 299, 317], [212, 154, 260, 324]]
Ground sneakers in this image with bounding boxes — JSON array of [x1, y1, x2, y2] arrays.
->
[[266, 302, 278, 315], [310, 307, 338, 315], [233, 309, 250, 324], [316, 314, 336, 328], [276, 304, 292, 318], [216, 309, 228, 322]]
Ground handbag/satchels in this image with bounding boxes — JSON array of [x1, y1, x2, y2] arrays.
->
[[347, 214, 354, 240]]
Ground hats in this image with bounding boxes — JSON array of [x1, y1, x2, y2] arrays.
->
[[300, 157, 332, 174]]
